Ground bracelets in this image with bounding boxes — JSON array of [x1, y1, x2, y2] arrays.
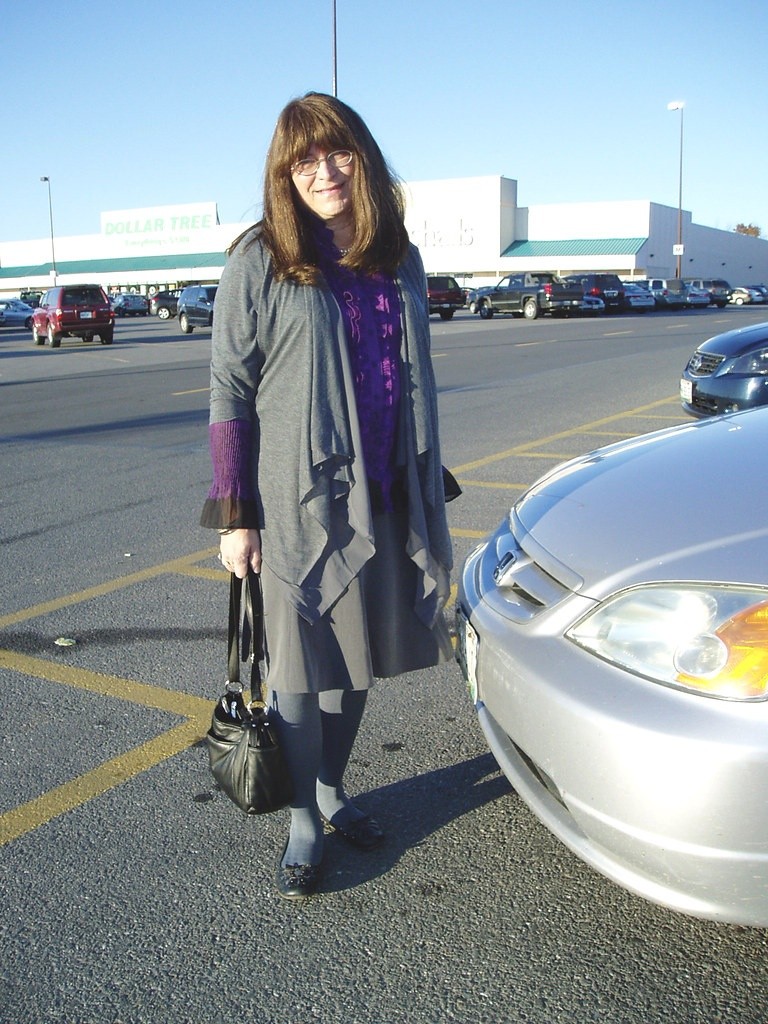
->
[[217, 529, 235, 534]]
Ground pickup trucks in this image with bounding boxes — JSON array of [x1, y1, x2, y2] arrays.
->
[[476, 272, 585, 320]]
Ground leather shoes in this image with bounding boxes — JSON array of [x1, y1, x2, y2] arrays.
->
[[313, 794, 385, 844], [275, 832, 329, 900]]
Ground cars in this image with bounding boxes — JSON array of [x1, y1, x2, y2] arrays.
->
[[449, 405, 768, 928], [110, 293, 148, 317], [0, 298, 35, 330], [459, 287, 476, 305], [728, 287, 763, 306], [743, 284, 768, 301], [679, 324, 767, 417], [622, 284, 656, 310], [686, 283, 711, 308]]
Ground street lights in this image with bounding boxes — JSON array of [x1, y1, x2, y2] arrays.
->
[[666, 100, 685, 279], [40, 175, 56, 286]]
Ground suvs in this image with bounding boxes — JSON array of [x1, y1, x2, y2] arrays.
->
[[176, 283, 219, 333], [561, 273, 629, 313], [683, 277, 734, 310], [29, 284, 115, 348], [150, 288, 186, 320], [16, 290, 47, 309], [635, 277, 688, 310], [427, 276, 465, 320]]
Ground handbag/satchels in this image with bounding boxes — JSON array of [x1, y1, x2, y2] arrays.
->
[[206, 564, 288, 819]]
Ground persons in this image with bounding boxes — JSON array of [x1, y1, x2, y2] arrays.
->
[[200, 94, 453, 899]]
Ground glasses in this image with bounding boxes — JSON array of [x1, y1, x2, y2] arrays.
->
[[288, 148, 355, 177]]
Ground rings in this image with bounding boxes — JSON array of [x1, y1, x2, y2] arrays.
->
[[218, 553, 223, 559]]
